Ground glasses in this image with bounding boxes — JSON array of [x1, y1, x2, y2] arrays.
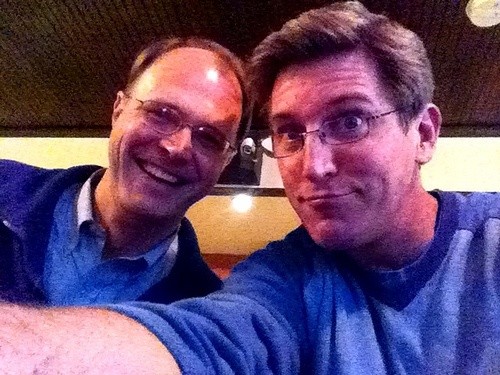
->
[[125, 95, 240, 156], [258, 105, 409, 160]]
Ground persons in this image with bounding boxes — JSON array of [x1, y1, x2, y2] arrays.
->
[[1, 0, 500, 375], [0, 35, 253, 304]]
